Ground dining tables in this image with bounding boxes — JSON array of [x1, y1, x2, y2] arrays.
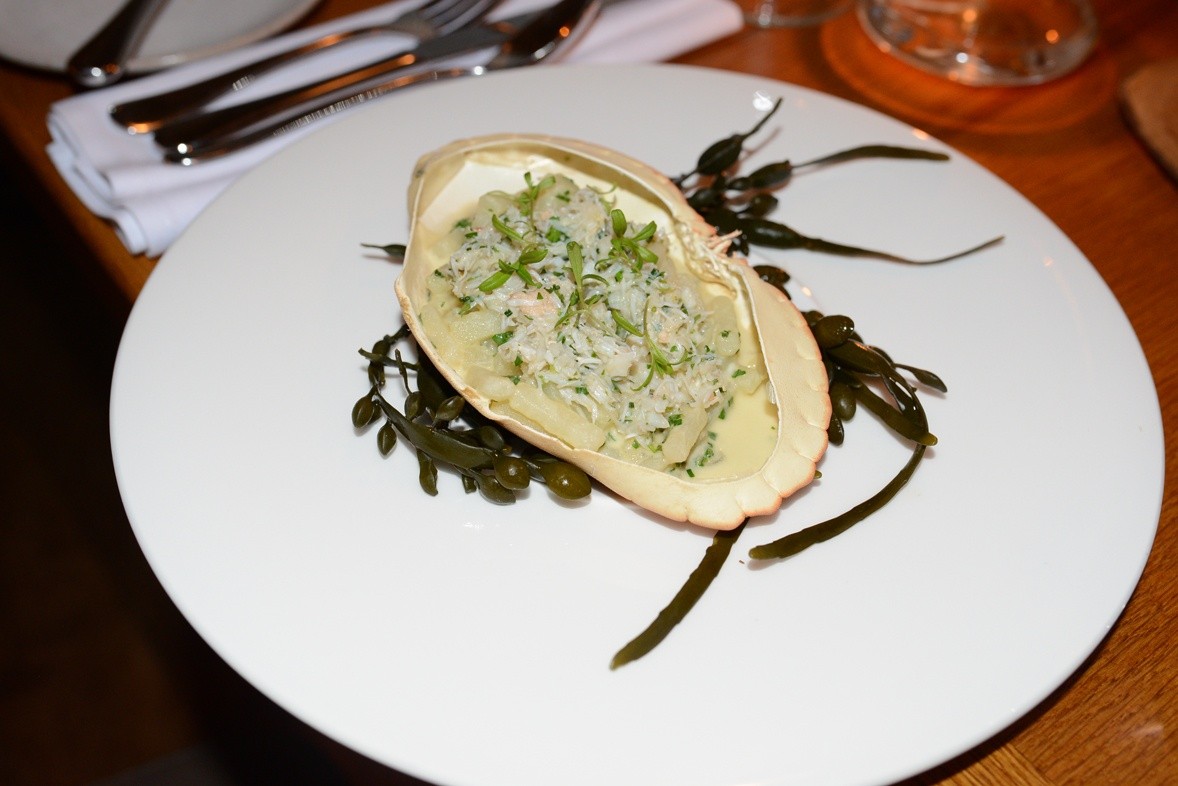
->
[[0, 1, 1177, 786]]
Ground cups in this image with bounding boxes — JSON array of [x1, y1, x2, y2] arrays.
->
[[858, 0, 1100, 84]]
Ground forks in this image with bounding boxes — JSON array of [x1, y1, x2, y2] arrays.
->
[[108, 0, 502, 135]]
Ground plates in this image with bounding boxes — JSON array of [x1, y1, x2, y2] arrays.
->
[[106, 63, 1161, 782], [821, 1, 1121, 138]]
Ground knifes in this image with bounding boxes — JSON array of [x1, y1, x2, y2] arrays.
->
[[153, 2, 557, 148]]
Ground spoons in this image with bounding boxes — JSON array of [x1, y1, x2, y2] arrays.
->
[[167, 0, 606, 168]]
